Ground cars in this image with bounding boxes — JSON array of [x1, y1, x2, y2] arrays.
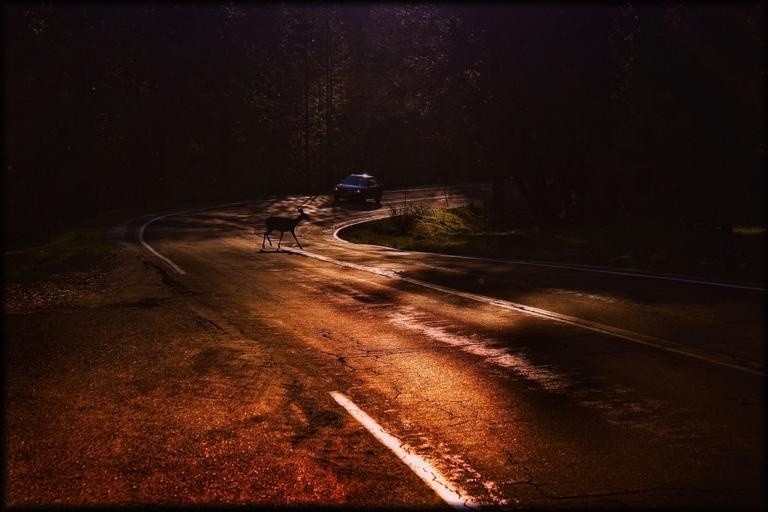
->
[[334, 172, 386, 207]]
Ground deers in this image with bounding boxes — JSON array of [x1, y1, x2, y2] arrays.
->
[[261, 206, 312, 253]]
